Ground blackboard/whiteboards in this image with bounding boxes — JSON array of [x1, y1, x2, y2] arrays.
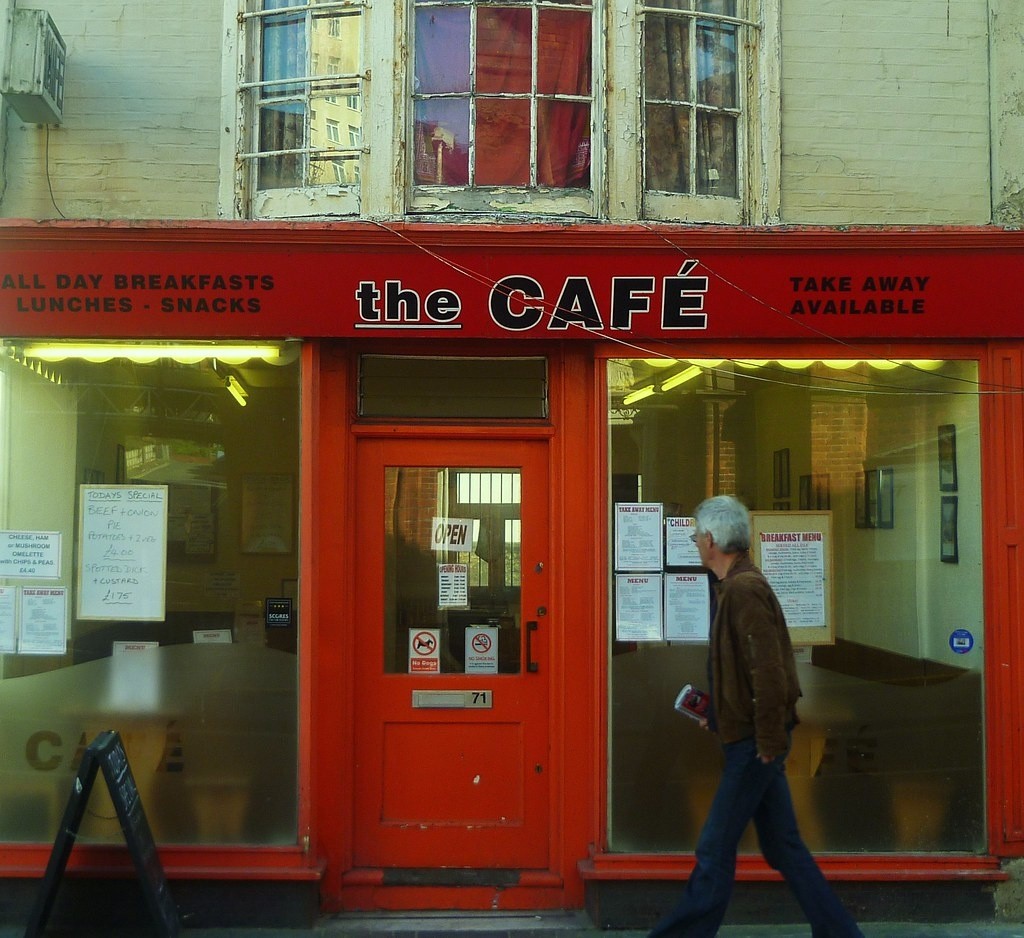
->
[[25, 728, 186, 938], [76, 484, 170, 621]]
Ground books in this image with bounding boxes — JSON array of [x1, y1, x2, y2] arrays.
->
[[674, 685, 710, 722]]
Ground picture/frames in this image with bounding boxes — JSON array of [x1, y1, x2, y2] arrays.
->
[[773, 501, 790, 510], [799, 474, 830, 510], [937, 424, 959, 492], [855, 468, 894, 530], [773, 448, 791, 498], [115, 444, 126, 484], [84, 468, 106, 484], [940, 496, 958, 564], [239, 472, 296, 557]]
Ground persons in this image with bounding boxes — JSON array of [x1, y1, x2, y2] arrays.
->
[[646, 495, 865, 938]]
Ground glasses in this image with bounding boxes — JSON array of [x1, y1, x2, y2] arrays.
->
[[689, 533, 708, 543]]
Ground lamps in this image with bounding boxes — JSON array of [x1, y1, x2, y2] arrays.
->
[[224, 374, 249, 407], [658, 365, 704, 392], [708, 168, 720, 195], [621, 383, 663, 404]]
